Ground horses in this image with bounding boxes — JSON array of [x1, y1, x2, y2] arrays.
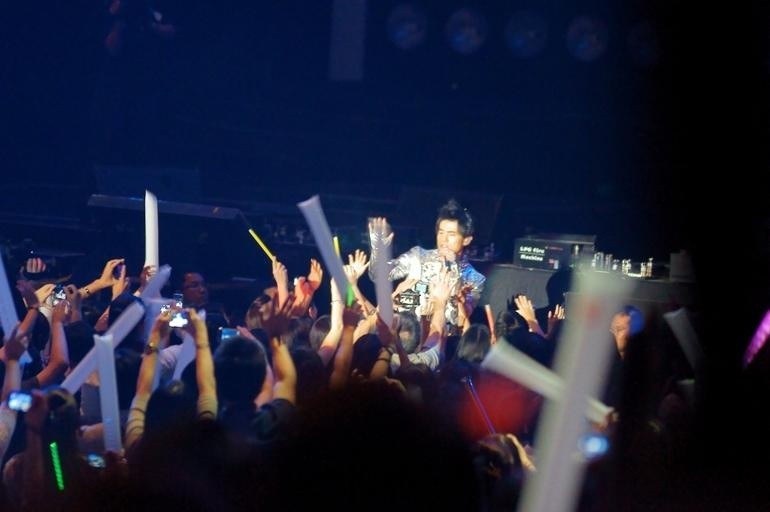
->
[[113, 263, 122, 279], [52, 288, 66, 300], [216, 327, 240, 341], [87, 453, 105, 471], [174, 293, 183, 306]]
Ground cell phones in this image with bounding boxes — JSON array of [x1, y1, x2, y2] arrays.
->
[[168, 309, 192, 328], [8, 390, 32, 413], [396, 281, 428, 307]]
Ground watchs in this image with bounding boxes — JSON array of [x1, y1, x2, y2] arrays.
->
[[144, 344, 158, 354]]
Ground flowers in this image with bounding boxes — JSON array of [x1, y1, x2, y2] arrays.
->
[[444, 255, 452, 272]]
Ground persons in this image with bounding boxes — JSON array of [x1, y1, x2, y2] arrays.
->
[[365, 196, 488, 320], [0, 252, 769, 512]]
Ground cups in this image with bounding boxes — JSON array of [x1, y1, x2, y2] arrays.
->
[[181, 280, 209, 289]]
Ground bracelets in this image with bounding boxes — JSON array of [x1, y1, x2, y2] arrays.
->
[[28, 306, 38, 311], [377, 358, 391, 363], [196, 342, 209, 349], [84, 286, 91, 297], [382, 344, 395, 351], [380, 346, 393, 355], [330, 299, 345, 305]]
